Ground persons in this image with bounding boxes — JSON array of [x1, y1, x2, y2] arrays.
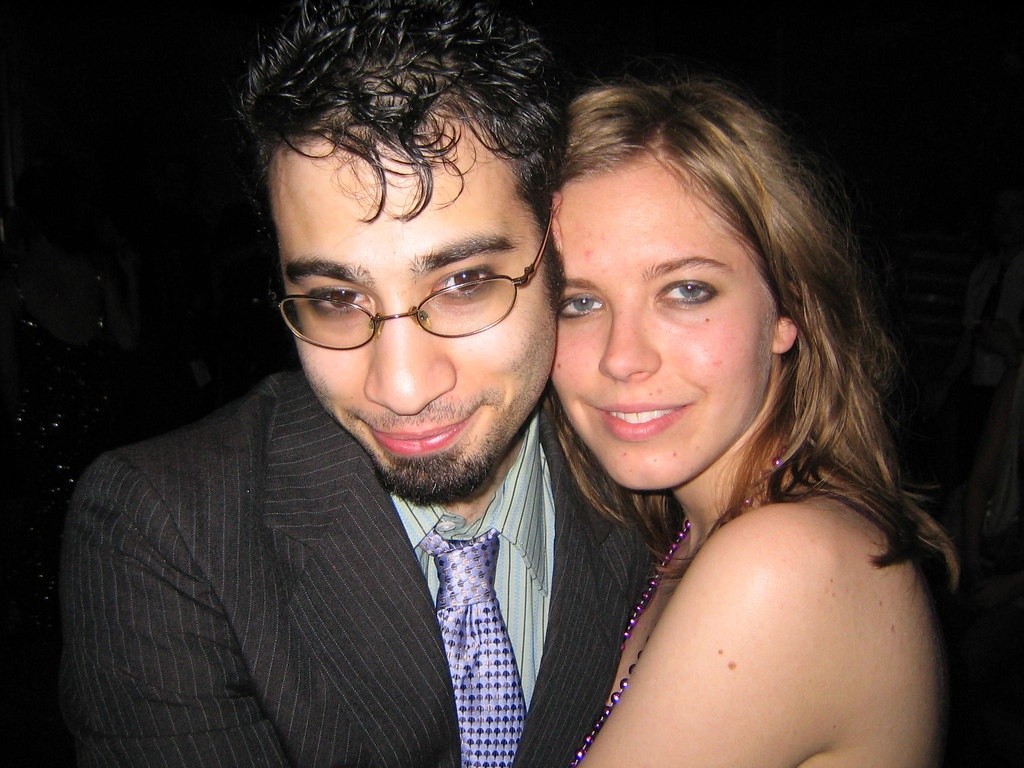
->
[[904, 172, 1024, 767], [548, 72, 962, 768], [60, 0, 685, 768], [0, 165, 143, 665]]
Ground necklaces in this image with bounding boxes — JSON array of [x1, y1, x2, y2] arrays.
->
[[570, 444, 789, 768]]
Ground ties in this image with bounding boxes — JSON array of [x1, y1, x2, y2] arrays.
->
[[970, 262, 1009, 352], [418, 528, 527, 768]]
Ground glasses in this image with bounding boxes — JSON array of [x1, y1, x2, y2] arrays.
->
[[266, 210, 553, 351]]
